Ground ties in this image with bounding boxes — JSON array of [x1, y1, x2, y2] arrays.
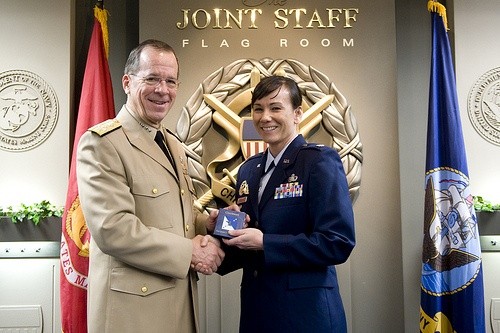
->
[[154, 130, 178, 180]]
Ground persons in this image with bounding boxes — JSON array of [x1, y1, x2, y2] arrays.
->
[[192, 76, 356, 333], [76, 40, 251, 333]]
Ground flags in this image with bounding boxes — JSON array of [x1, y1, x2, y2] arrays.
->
[[419, 0, 485, 333], [60, 4, 116, 333]]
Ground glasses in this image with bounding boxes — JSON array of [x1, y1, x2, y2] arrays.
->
[[129, 73, 180, 90]]
